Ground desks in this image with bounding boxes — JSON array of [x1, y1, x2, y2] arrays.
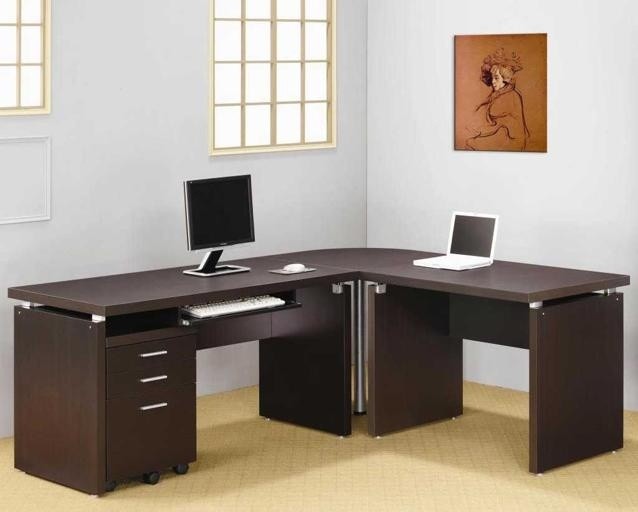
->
[[268, 247, 630, 477]]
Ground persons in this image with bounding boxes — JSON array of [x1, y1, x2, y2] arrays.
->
[[464, 46, 532, 152]]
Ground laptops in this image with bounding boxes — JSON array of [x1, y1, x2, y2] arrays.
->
[[413, 211, 499, 271]]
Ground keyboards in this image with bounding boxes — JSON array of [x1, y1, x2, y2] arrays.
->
[[180, 294, 285, 319]]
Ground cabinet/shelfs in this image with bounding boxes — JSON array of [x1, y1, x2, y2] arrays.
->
[[8, 249, 359, 497]]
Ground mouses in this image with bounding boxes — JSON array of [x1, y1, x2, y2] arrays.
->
[[284, 262, 306, 273]]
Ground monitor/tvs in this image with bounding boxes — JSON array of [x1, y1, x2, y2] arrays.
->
[[182, 174, 255, 277]]
